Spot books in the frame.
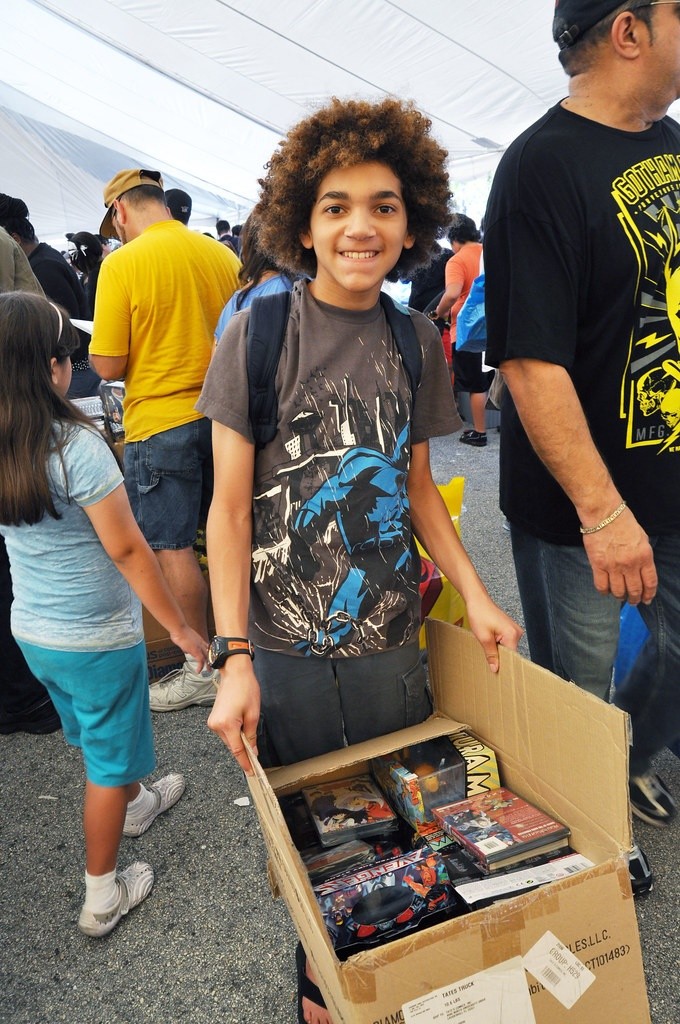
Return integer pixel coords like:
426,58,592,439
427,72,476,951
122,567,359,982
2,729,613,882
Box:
429,785,571,871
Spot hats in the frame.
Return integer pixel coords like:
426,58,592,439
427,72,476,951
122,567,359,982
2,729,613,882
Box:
216,220,229,235
68,230,102,270
553,0,628,51
98,168,164,241
165,189,192,214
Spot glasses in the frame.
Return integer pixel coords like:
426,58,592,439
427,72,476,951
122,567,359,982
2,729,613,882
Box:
105,242,112,246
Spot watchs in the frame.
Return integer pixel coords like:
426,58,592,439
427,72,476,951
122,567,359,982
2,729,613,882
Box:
206,635,255,669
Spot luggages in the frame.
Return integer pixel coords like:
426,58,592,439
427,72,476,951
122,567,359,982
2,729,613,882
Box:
457,389,501,432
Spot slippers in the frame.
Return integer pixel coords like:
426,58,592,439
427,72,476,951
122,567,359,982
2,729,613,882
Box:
295,939,328,1024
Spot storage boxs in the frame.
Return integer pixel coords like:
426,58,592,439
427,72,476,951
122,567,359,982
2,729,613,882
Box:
369,732,502,832
240,614,654,1024
143,568,216,686
315,842,458,956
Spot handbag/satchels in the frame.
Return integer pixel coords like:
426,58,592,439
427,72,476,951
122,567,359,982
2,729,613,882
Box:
455,274,488,353
417,473,474,649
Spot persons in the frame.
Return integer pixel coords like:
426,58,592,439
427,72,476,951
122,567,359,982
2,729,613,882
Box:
87,168,249,713
479,0,680,899
0,291,209,939
165,188,244,258
409,212,499,448
0,192,113,737
195,93,525,1024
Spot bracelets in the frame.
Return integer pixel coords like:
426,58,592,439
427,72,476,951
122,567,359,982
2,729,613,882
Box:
580,502,626,535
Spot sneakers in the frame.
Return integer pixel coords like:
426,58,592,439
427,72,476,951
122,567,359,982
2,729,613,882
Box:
628,766,676,898
147,662,219,712
0,694,63,736
122,773,185,838
78,861,155,938
459,431,488,447
